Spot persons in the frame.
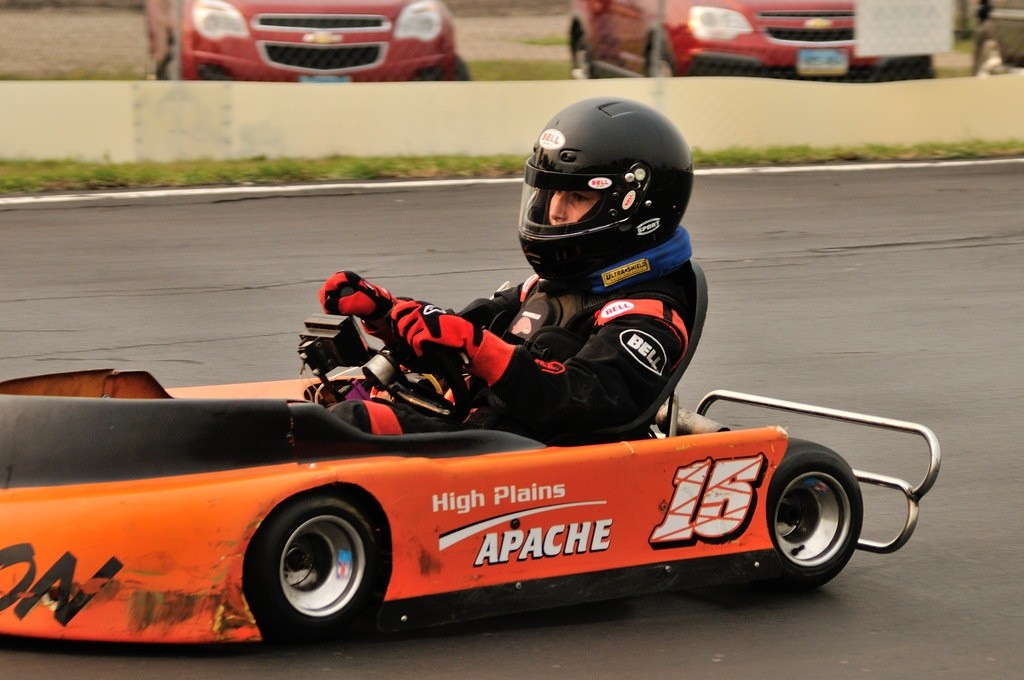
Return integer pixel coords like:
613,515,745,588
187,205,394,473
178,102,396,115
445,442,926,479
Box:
310,98,693,443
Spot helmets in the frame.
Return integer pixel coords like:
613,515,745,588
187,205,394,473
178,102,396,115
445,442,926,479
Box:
516,98,693,279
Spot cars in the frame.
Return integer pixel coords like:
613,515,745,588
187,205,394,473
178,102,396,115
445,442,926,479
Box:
142,0,474,84
972,0,1021,76
565,1,935,78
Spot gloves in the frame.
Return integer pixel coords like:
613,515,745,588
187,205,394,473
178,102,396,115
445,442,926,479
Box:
318,269,484,361
